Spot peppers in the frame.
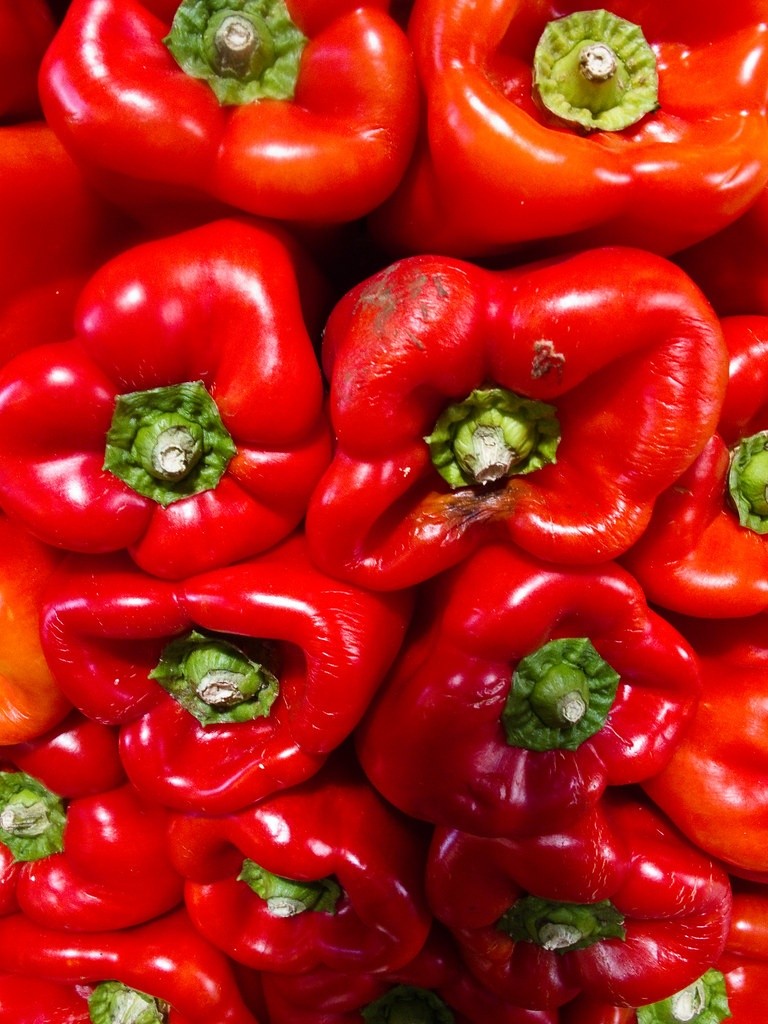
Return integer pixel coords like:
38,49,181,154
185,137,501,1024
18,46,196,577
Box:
0,0,768,1024
